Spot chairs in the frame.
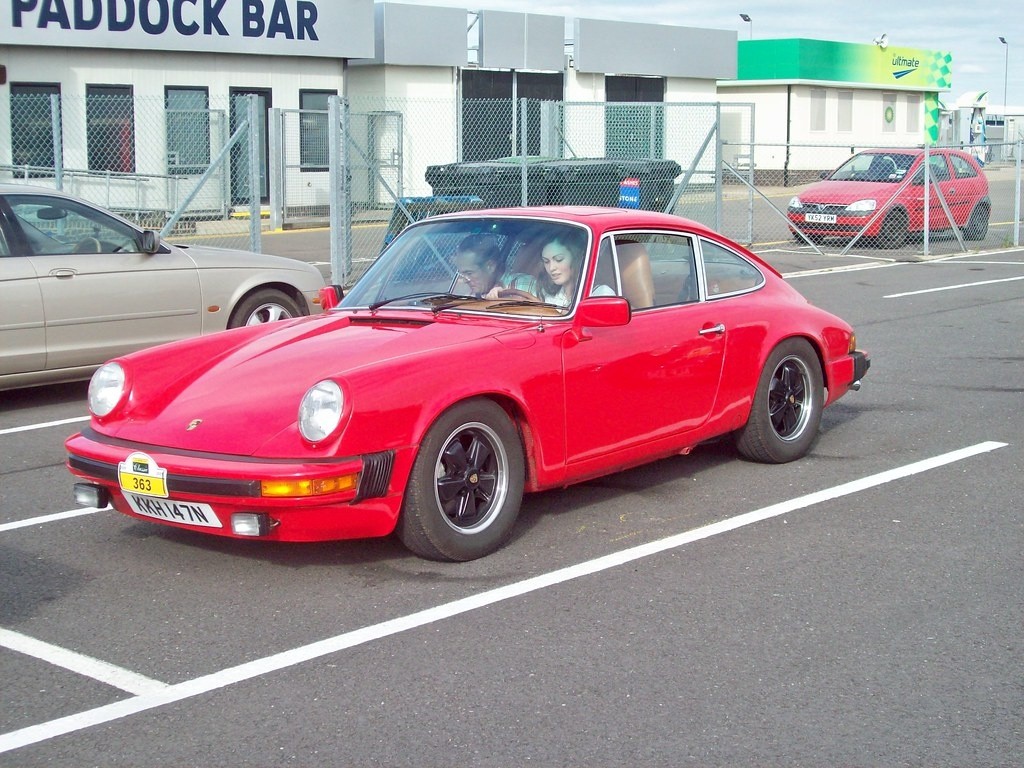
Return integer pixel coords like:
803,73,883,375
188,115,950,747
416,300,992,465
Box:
594,239,655,308
511,243,544,280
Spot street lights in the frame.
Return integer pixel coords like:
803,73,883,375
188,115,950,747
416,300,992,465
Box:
739,14,753,41
998,35,1009,107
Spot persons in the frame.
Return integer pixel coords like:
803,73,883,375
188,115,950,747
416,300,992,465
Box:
485,232,617,315
457,234,539,299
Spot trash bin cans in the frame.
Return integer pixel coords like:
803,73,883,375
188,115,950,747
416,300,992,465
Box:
426,154,683,273
383,195,488,277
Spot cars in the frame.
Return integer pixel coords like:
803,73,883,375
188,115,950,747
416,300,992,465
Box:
0,180,326,392
68,203,872,558
787,148,990,249
